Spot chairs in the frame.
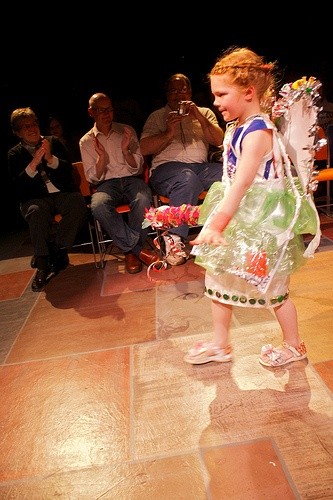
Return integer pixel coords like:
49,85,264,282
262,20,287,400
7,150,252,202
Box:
61,124,333,268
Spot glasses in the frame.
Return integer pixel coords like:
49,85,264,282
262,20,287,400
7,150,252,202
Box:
96,107,113,113
19,122,39,131
170,87,190,94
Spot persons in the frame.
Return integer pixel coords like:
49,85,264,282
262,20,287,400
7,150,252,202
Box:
141,73,225,267
145,48,323,366
47,114,79,162
7,106,83,290
79,92,166,274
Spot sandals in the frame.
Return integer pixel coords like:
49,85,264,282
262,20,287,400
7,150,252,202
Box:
183,341,235,365
257,341,308,366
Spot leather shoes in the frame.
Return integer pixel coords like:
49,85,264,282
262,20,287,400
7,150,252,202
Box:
124,251,143,274
136,237,165,270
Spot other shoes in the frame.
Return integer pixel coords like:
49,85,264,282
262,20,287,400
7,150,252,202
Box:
32,268,59,290
31,244,60,270
155,232,186,266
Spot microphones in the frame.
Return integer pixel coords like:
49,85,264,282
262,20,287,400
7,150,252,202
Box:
178,102,186,116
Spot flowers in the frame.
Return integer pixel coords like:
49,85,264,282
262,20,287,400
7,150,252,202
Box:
141,203,200,231
